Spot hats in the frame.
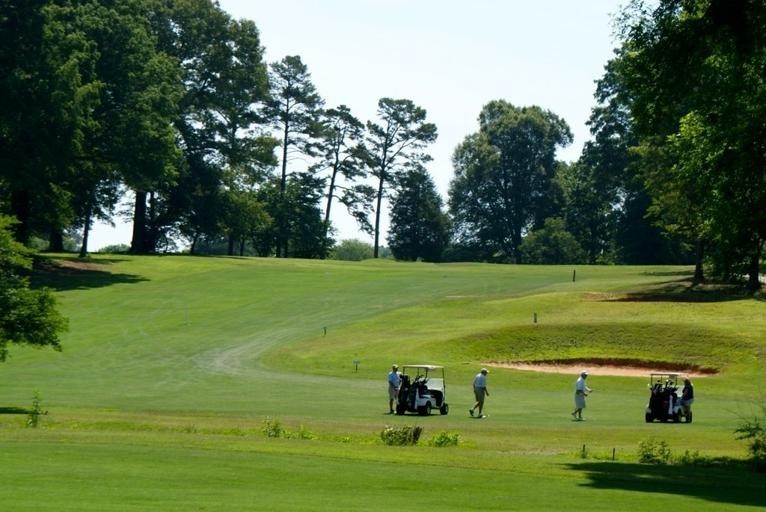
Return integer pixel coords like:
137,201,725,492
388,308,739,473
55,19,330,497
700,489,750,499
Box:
393,365,398,368
481,368,488,373
581,370,588,375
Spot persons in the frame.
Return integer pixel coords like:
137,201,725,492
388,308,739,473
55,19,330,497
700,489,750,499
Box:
468,369,490,418
570,371,594,422
387,364,403,414
680,377,693,421
